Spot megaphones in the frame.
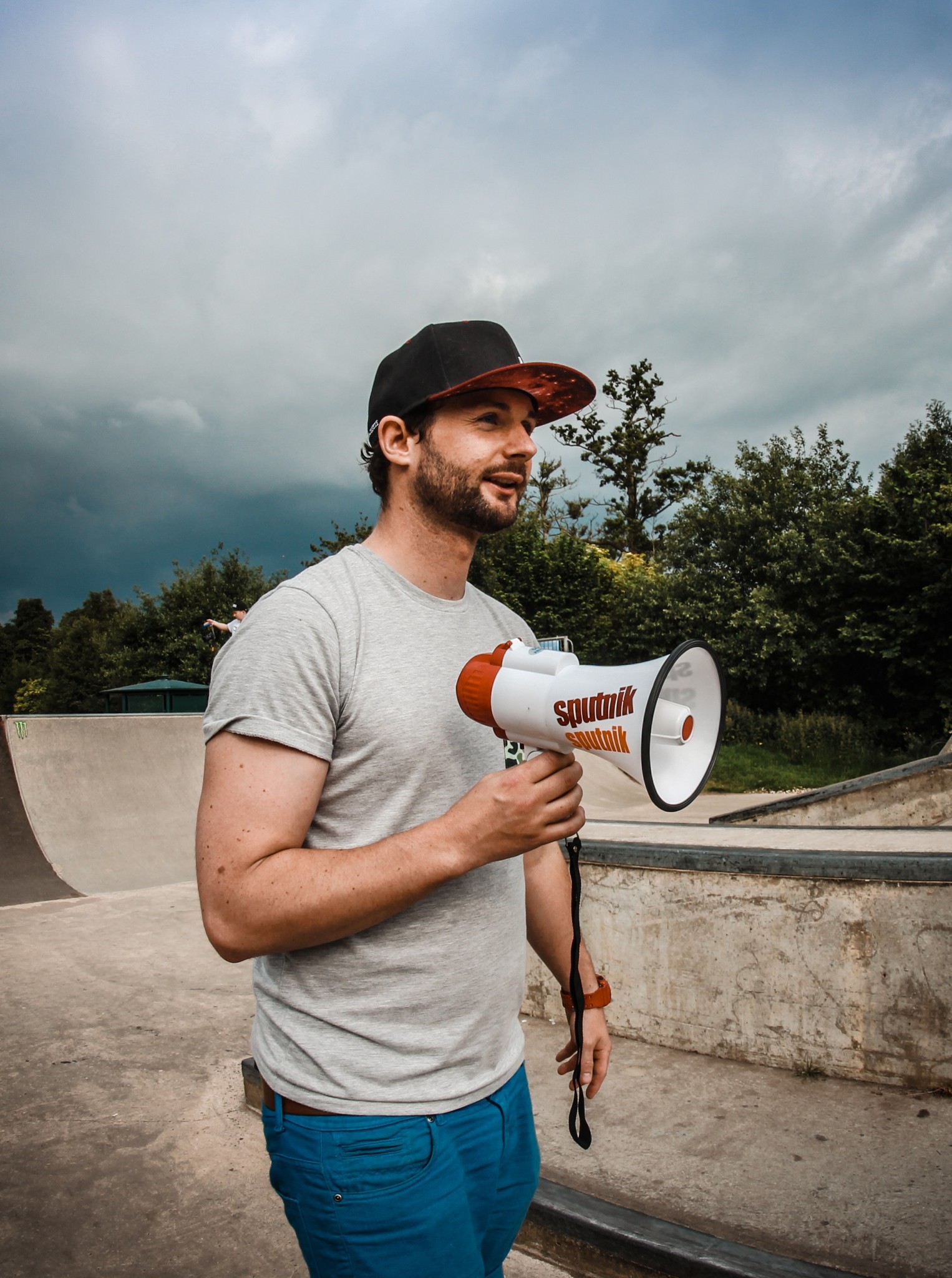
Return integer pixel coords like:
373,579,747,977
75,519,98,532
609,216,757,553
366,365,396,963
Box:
456,636,725,812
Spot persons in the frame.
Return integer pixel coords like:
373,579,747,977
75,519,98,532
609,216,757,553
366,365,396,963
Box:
206,600,249,636
195,320,613,1278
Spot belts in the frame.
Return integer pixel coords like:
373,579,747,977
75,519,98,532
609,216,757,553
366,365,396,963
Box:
262,1077,340,1116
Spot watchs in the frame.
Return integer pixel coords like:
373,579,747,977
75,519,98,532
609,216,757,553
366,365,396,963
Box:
560,973,612,1010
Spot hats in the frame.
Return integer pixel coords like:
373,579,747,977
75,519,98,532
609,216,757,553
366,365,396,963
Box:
367,321,596,455
